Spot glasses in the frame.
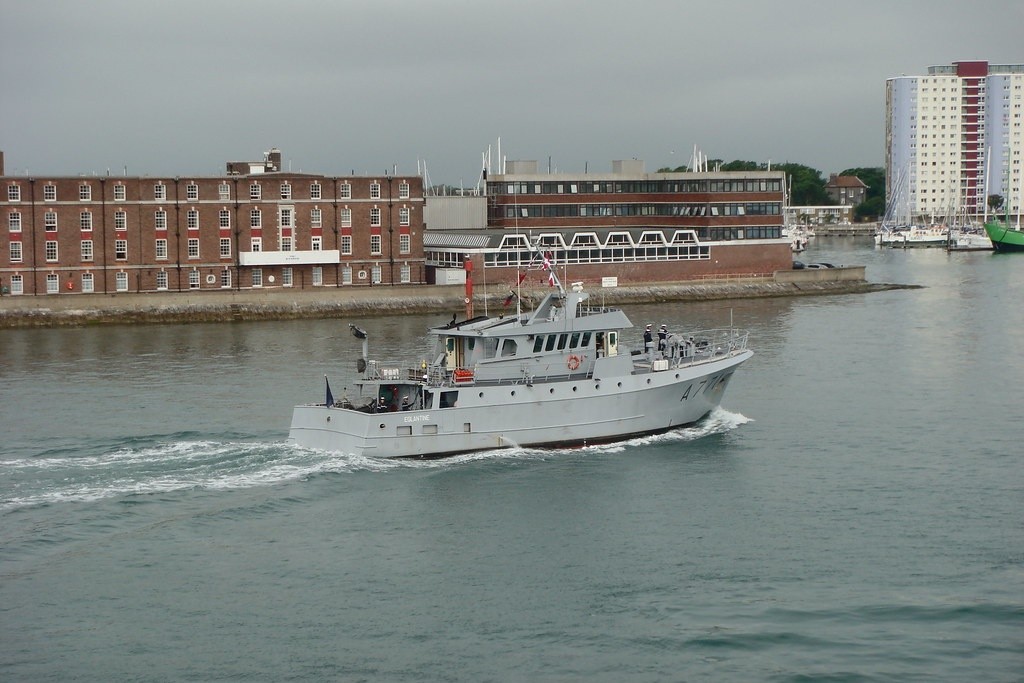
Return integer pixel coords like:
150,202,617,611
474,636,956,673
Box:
406,399,408,400
380,399,385,400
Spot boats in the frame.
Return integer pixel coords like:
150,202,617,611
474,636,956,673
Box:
982,148,1024,252
781,216,815,252
872,146,993,251
288,184,755,459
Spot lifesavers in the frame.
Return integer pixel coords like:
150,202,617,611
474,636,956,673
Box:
567,356,580,370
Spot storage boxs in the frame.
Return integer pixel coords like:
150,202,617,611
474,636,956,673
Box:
381,369,399,380
653,360,668,371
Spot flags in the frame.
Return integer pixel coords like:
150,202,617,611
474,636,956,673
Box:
516,274,525,286
549,271,554,287
503,296,512,306
325,378,333,408
543,249,550,271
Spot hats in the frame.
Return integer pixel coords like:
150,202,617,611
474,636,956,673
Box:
661,325,666,326
646,324,653,327
403,396,408,399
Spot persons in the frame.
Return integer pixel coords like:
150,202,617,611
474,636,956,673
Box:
658,324,668,351
643,324,652,353
